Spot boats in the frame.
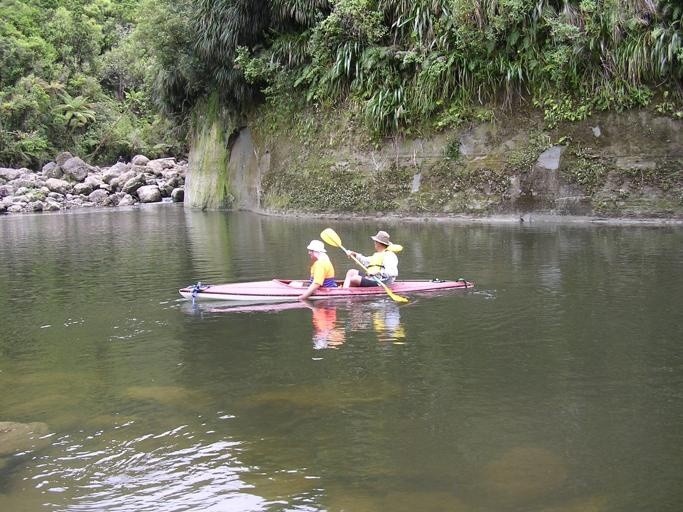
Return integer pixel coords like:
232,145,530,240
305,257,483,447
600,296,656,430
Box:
178,278,476,304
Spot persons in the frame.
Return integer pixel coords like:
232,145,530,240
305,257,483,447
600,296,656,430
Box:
341,230,404,289
295,239,336,302
349,298,405,345
301,299,337,351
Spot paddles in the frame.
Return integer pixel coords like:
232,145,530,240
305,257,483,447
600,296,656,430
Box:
320,227,408,302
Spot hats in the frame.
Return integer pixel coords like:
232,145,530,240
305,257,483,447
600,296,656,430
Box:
307,240,327,253
370,231,390,245
